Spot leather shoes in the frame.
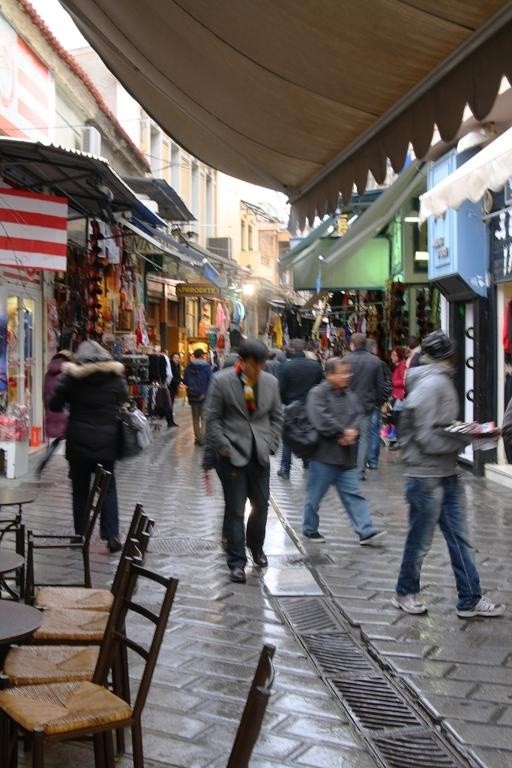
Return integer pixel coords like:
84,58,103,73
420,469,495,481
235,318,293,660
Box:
71,536,88,547
247,542,267,565
230,567,245,582
277,468,289,479
109,535,121,551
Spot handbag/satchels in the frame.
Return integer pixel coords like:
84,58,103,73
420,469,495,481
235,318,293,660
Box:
278,386,328,459
115,400,150,454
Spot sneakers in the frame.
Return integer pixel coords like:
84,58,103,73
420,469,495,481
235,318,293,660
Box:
359,530,386,545
391,593,428,614
303,531,324,543
455,597,507,617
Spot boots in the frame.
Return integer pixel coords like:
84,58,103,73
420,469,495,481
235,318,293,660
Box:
33,446,52,482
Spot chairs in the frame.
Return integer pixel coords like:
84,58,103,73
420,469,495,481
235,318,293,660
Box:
32,518,154,708
28,464,112,588
3,538,142,754
1,558,179,768
225,643,277,767
32,501,147,609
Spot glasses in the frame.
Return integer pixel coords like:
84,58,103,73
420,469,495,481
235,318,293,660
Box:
328,370,353,377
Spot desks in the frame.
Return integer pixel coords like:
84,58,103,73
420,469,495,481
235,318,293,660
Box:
1,546,25,600
0,599,44,643
1,488,34,526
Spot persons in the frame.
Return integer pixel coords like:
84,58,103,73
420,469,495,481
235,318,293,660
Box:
193,327,346,360
279,338,323,480
302,358,387,542
186,351,214,449
200,339,284,582
394,332,508,618
340,335,384,479
48,342,134,553
166,354,179,405
367,340,391,470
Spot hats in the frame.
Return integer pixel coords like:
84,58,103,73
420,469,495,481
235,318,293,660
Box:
420,330,459,360
73,340,112,362
193,349,206,355
